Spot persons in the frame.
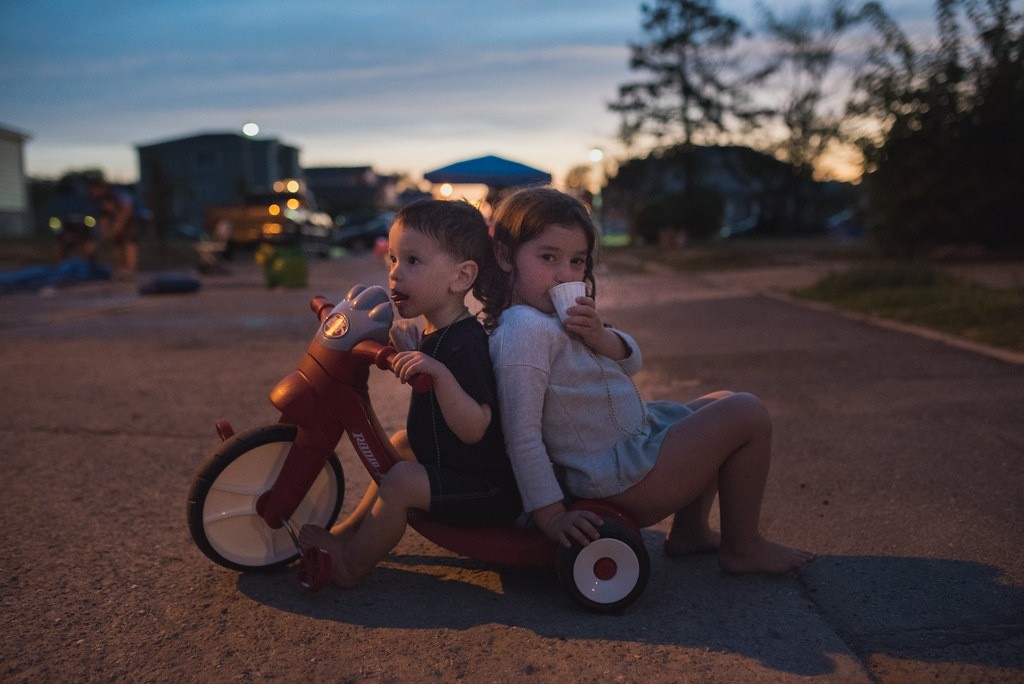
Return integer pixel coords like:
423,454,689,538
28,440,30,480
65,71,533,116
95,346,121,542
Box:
474,187,817,573
298,199,523,589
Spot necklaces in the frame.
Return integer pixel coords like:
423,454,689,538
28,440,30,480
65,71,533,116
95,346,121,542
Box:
430,306,468,469
551,312,647,434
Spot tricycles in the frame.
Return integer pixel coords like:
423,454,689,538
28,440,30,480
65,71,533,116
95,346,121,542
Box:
181,284,651,616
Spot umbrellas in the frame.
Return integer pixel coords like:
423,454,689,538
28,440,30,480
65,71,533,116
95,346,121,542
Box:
424,156,552,222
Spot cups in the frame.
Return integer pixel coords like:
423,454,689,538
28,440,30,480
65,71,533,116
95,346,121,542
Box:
548,281,587,324
